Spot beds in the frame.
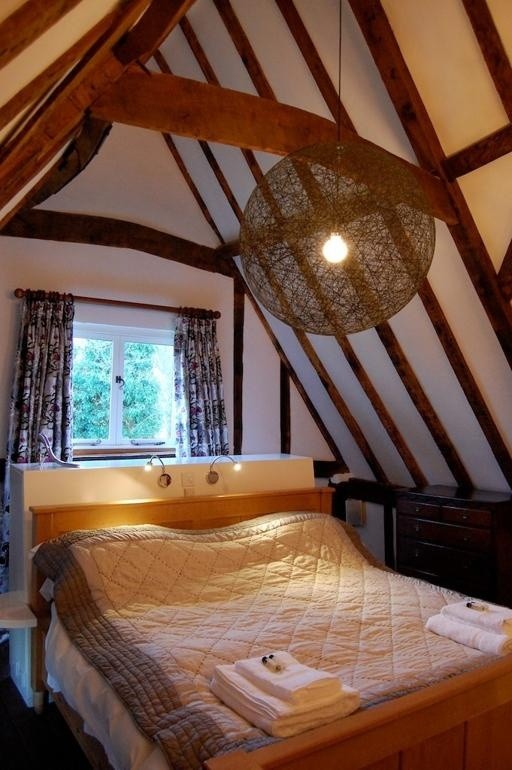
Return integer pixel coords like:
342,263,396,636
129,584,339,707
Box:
30,487,512,769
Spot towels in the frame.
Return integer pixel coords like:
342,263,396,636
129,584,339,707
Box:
441,600,512,633
209,653,360,738
425,600,512,657
234,650,341,704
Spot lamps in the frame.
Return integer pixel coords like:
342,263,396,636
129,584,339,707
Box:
144,455,172,488
238,0,436,336
206,455,242,484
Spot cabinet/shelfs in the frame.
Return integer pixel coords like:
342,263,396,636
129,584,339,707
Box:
394,485,512,605
